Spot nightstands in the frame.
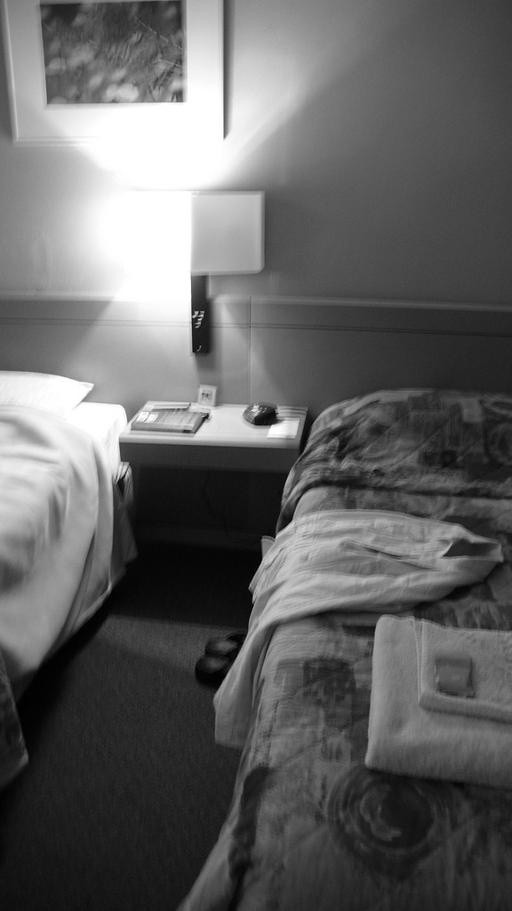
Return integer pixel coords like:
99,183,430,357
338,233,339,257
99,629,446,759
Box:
119,401,309,560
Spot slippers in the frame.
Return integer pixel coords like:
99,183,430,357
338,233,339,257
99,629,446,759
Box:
196,632,248,688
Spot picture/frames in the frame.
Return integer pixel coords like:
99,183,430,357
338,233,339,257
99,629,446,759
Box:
1,0,225,145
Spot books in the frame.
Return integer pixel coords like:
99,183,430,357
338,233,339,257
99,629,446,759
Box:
129,409,210,435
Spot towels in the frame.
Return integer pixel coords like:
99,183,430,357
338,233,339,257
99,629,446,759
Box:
363,612,511,790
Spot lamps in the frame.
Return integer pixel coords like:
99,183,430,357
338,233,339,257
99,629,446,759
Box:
190,192,266,356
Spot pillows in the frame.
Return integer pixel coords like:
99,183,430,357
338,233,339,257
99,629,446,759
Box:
1,369,95,422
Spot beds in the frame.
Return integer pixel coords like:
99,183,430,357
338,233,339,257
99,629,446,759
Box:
234,387,512,910
0,367,127,779
